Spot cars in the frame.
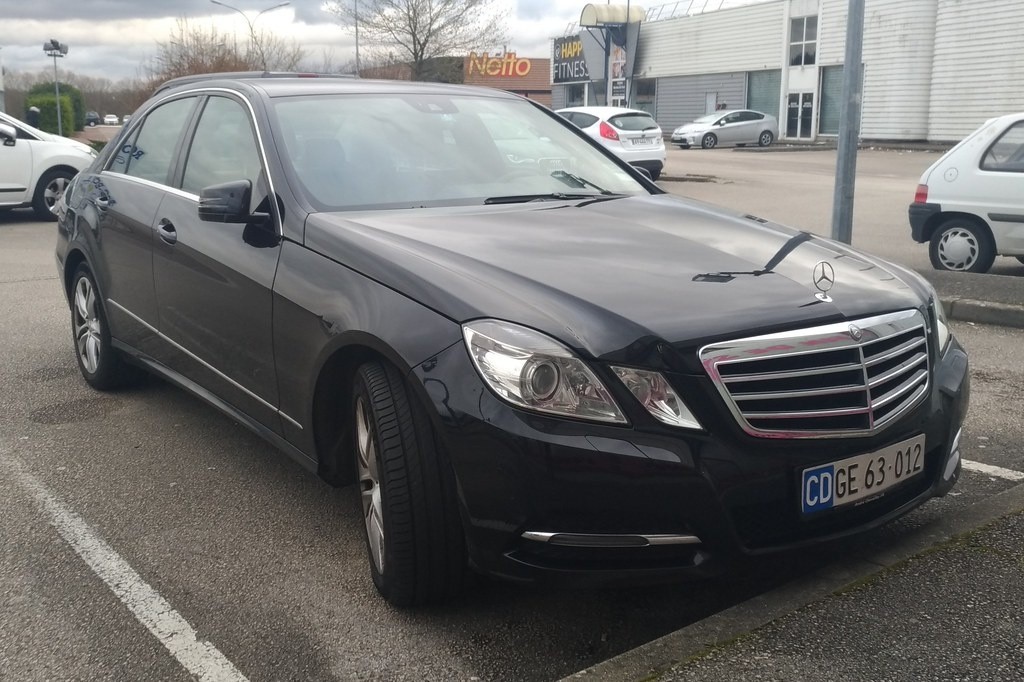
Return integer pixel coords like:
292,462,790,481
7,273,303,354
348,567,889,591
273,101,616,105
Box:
57,70,970,611
84,110,100,126
908,111,1024,276
103,114,119,125
0,109,101,222
669,109,780,150
554,106,666,182
123,114,130,123
